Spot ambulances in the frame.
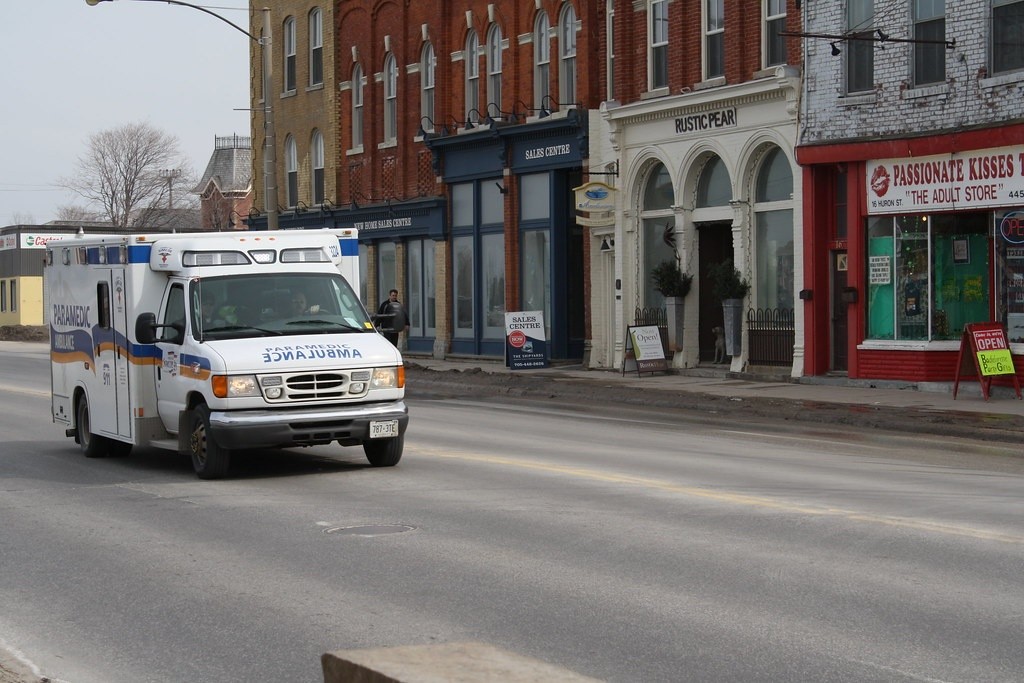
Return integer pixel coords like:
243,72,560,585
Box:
41,225,409,482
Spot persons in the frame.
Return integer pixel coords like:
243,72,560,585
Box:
374,289,410,348
176,293,232,333
287,291,322,318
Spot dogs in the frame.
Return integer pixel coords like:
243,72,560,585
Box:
712,327,731,364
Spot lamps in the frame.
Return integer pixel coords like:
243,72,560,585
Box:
465,109,508,130
830,29,890,56
670,205,692,214
246,207,262,226
293,201,309,220
729,199,751,210
485,103,526,125
539,95,582,118
417,116,456,136
601,234,614,250
441,114,479,137
350,192,389,211
389,191,426,217
511,101,552,125
78,228,85,238
319,198,352,216
229,211,250,228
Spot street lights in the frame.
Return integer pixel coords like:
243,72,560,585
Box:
83,0,280,231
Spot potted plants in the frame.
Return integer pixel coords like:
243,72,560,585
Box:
708,257,752,356
651,259,695,353
